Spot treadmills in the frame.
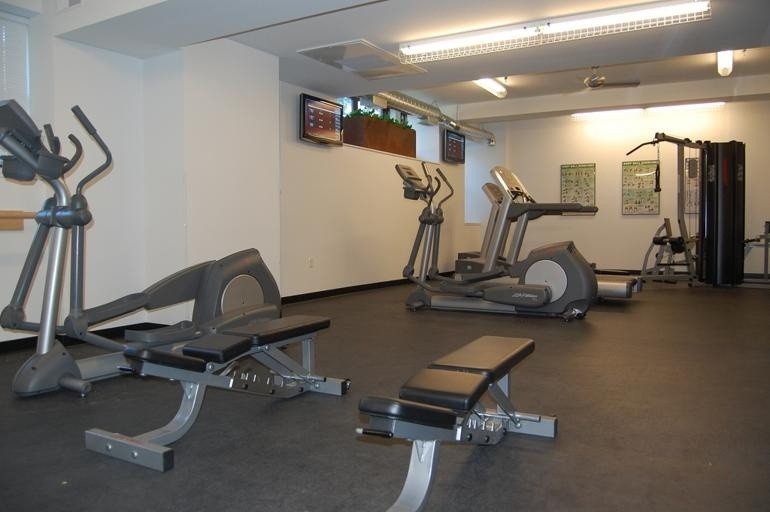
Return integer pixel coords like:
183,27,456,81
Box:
454,178,643,303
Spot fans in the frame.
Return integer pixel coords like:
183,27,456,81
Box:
564,74,641,96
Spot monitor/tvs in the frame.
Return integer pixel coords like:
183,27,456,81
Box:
444,128,465,163
300,93,343,146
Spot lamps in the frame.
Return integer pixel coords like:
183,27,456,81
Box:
400,0,710,55
473,78,507,99
716,50,732,76
